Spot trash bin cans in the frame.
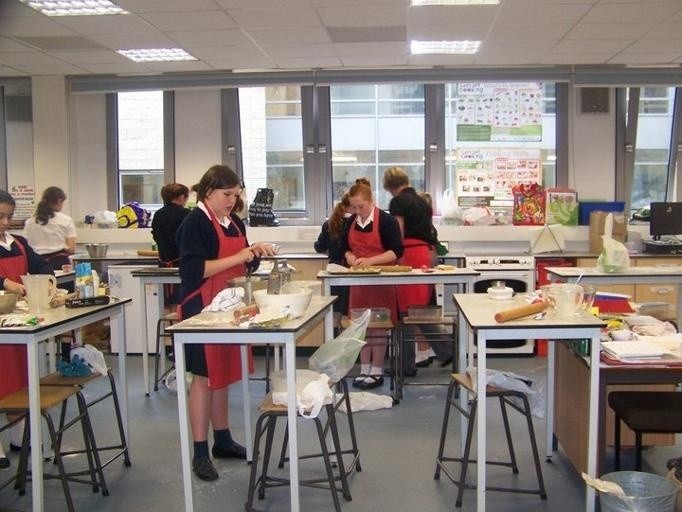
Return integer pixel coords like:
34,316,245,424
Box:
579,469,681,511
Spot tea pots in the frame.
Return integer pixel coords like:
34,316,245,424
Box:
19,275,57,312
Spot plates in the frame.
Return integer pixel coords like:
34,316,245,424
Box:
327,268,382,275
129,267,181,276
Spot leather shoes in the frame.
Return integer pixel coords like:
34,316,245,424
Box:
212,444,247,462
416,360,430,367
0,457,11,471
9,441,45,455
385,367,418,378
191,459,220,484
352,372,366,387
359,376,385,390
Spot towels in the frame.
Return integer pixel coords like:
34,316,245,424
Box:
199,286,245,313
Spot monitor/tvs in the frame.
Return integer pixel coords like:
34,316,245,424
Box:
649,201,682,241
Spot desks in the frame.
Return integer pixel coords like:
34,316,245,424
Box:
534,251,682,320
48,270,101,375
452,293,608,512
318,269,481,409
438,250,467,317
163,296,339,511
0,291,132,512
72,254,159,270
545,266,682,333
555,340,682,511
130,267,293,397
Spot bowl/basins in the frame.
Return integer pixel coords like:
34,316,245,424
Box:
227,276,262,300
0,290,21,314
85,243,109,258
539,284,597,317
253,289,313,318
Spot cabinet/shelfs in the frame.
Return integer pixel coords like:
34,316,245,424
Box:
259,260,329,347
108,265,167,354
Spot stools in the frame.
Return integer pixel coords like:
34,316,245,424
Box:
608,391,682,472
0,387,100,512
39,368,132,494
245,378,361,511
154,313,181,391
398,316,458,400
340,315,400,403
435,374,547,508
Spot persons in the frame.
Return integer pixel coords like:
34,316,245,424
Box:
315,190,352,338
382,167,454,377
176,165,275,480
336,178,404,390
151,183,192,361
415,194,437,367
24,187,77,364
0,191,57,471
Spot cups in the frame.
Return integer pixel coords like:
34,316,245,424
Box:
491,280,507,288
